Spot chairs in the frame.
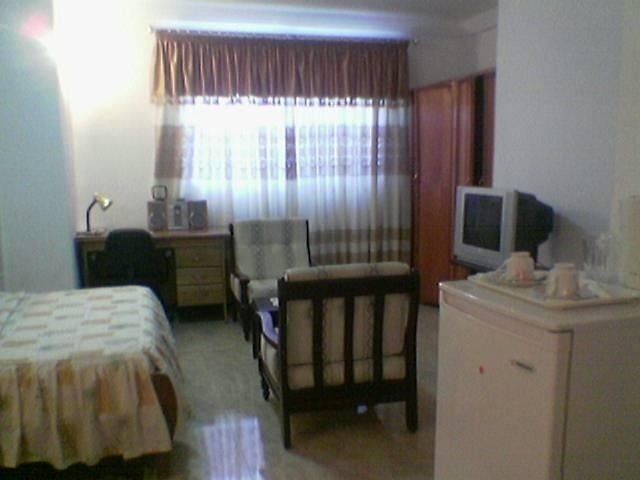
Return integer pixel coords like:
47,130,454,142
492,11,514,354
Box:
86,228,168,301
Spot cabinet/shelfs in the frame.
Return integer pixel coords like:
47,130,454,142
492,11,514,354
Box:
73,224,229,321
228,217,312,358
410,68,496,306
246,261,421,450
0,285,183,471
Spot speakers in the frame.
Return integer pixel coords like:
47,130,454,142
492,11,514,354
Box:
146,200,168,230
187,200,209,231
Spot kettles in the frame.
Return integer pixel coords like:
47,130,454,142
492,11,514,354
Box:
609,194,640,291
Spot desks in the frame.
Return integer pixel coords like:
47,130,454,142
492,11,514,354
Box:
251,298,281,358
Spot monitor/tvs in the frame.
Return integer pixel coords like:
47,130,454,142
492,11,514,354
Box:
450,185,553,274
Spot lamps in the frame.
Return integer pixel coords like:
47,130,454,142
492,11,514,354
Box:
80,193,113,235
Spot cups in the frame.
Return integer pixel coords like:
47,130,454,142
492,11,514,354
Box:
497,251,536,284
545,262,579,300
579,231,616,282
